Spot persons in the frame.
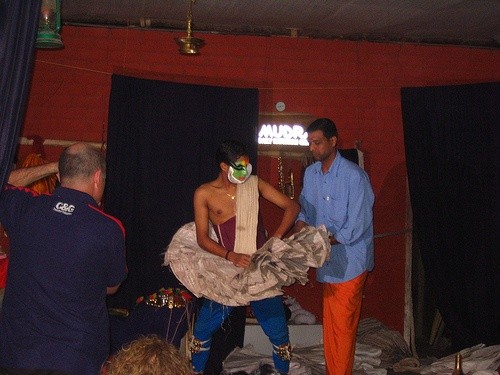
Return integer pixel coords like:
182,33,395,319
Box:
101,336,193,375
293,117,377,375
0,142,128,375
165,138,331,375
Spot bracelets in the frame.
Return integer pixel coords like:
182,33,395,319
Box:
225,250,232,260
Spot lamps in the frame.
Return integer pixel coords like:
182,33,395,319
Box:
36,0,64,50
